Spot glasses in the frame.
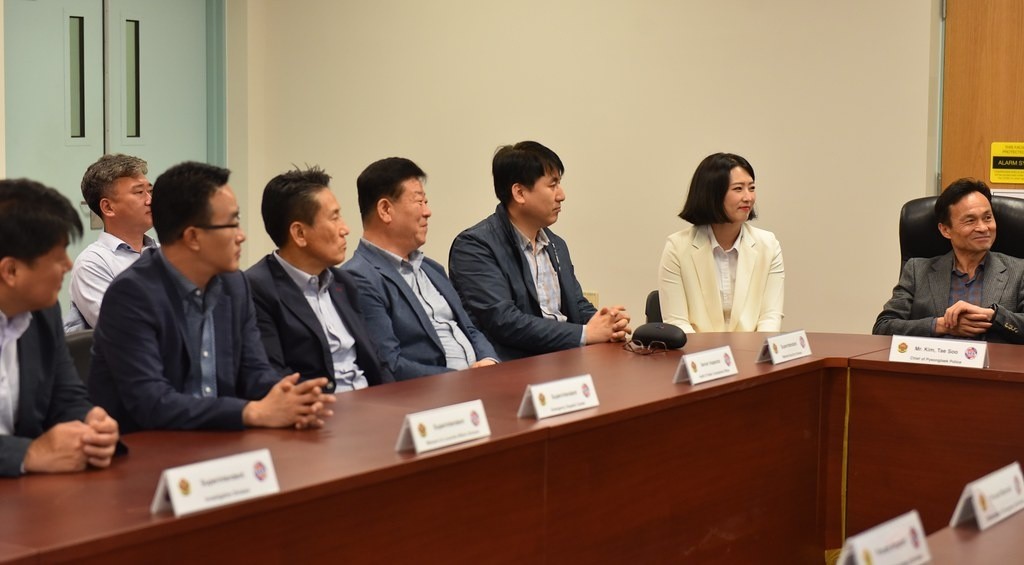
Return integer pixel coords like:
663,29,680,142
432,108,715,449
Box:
176,217,242,238
622,340,669,355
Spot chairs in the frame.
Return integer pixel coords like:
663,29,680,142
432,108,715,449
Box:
898,195,1024,278
65,330,92,387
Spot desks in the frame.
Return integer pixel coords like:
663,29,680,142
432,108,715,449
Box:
1,331,1024,564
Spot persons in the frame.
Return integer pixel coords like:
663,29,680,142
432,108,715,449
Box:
449,140,631,362
872,178,1024,345
64,153,161,334
659,153,784,333
0,177,128,478
340,156,503,381
85,160,337,435
244,162,394,396
